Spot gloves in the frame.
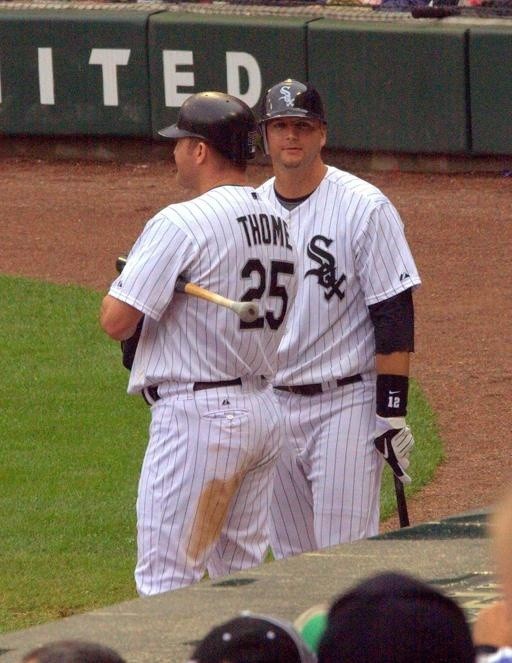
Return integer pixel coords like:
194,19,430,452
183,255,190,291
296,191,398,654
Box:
371,411,417,490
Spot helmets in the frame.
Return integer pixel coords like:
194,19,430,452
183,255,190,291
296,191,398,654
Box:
253,76,330,128
156,89,258,161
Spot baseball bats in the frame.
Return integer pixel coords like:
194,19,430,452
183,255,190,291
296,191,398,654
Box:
113,249,258,322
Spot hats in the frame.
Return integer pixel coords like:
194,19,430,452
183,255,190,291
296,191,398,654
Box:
291,601,332,656
317,569,476,663
188,609,301,662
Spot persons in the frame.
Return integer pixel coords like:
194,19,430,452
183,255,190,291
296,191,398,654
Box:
98,90,297,599
253,79,421,555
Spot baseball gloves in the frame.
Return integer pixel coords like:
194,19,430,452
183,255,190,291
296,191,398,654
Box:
121,316,145,370
